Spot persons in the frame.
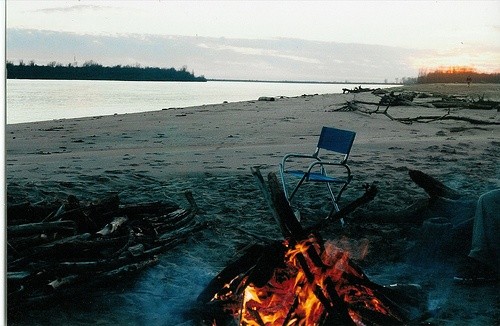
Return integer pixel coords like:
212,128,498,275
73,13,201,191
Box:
463,188,500,282
54,195,97,235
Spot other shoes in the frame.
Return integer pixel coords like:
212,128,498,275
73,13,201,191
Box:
454,261,497,282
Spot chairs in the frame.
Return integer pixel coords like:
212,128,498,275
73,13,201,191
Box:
278,126,357,230
